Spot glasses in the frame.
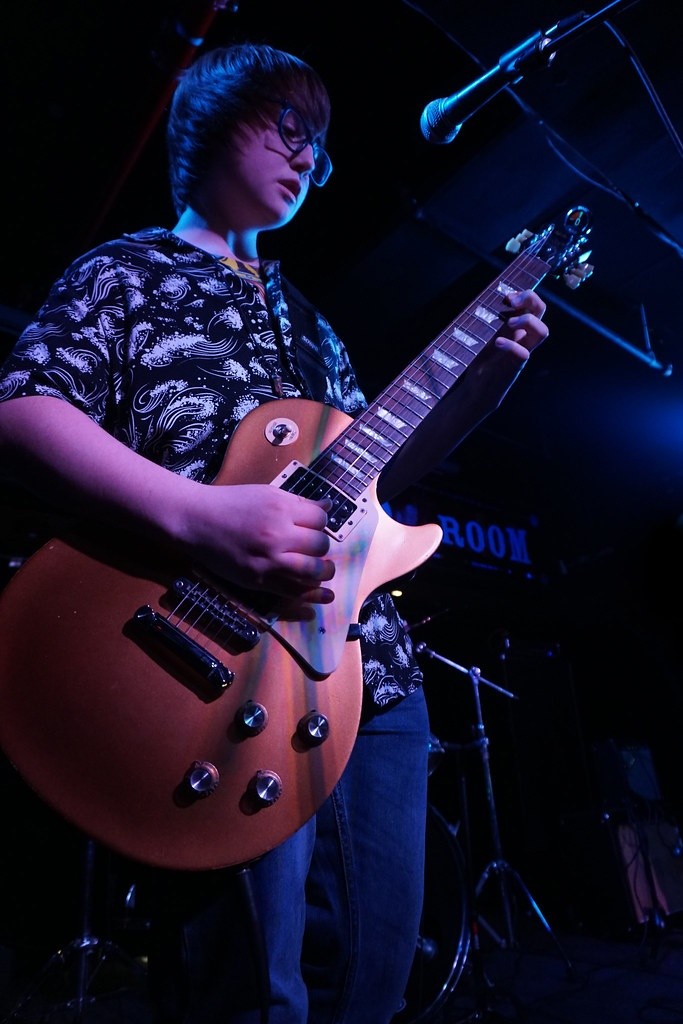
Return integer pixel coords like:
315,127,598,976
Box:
272,108,334,186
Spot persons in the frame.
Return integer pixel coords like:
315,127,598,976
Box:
0,43,548,1024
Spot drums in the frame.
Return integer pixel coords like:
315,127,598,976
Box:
147,801,473,1024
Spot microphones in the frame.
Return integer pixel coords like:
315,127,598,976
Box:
420,9,590,144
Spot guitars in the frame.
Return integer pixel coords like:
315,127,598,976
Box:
0,194,601,902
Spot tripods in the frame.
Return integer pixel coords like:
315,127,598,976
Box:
0,835,154,1024
413,641,577,975
